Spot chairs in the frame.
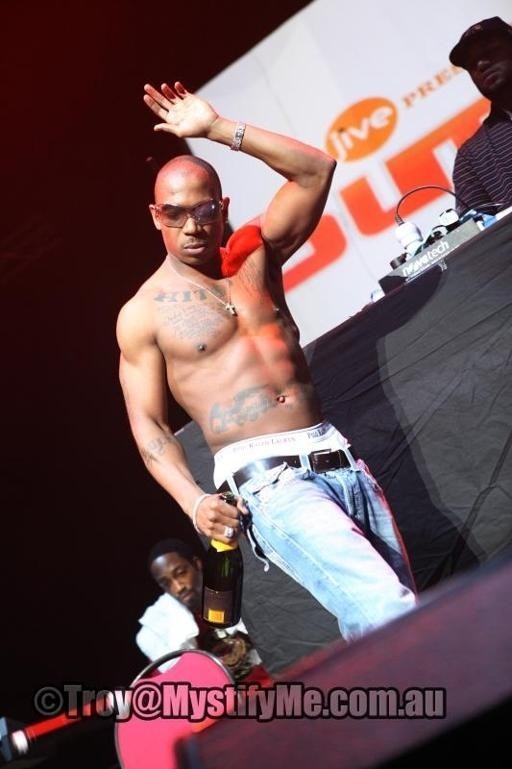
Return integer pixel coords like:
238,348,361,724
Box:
114,649,243,769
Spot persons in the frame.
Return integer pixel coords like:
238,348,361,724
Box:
133,535,274,710
448,13,512,220
114,76,425,642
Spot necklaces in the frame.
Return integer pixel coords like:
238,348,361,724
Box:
164,250,238,317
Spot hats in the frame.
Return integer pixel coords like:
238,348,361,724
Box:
449,16,512,66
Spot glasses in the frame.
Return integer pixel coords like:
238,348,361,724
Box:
156,199,223,228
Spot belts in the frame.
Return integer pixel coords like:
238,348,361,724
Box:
216,445,360,500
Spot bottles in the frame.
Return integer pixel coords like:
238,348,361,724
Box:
200,491,244,629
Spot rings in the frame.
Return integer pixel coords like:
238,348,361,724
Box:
223,526,235,539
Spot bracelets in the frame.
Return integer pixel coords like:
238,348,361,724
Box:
228,118,246,153
191,493,211,536
